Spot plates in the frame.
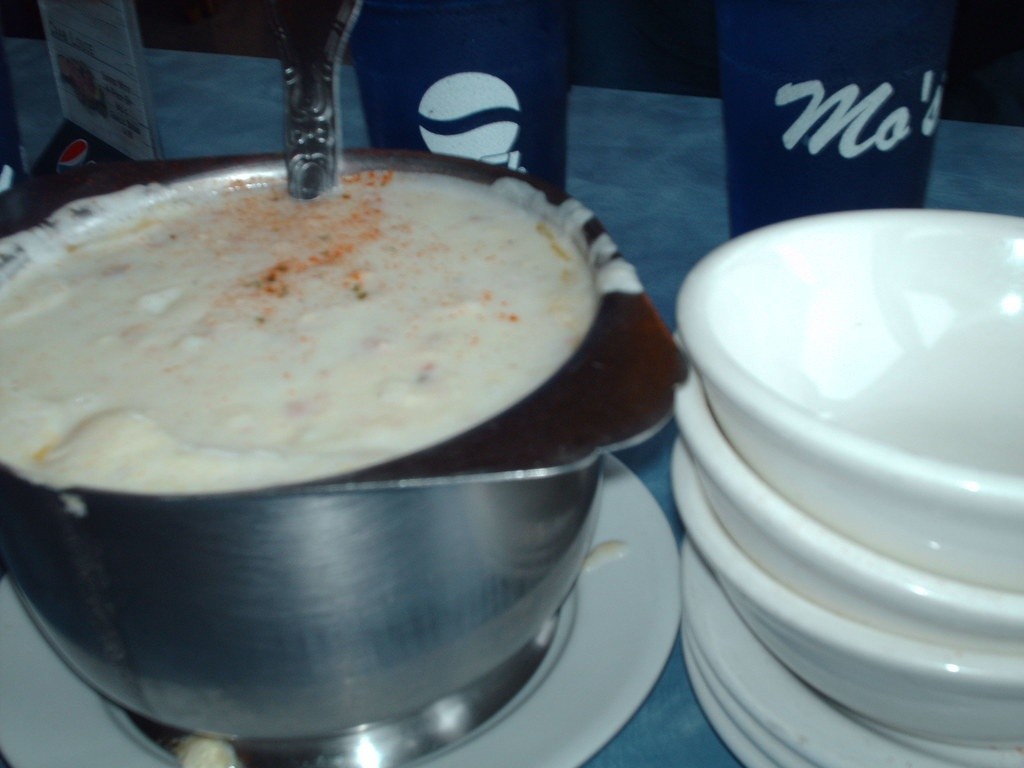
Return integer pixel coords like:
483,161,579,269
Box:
0,450,680,768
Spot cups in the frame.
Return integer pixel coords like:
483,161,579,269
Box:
0,155,683,751
714,0,953,236
349,0,569,195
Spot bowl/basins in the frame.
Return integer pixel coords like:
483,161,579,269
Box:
678,204,1024,768
